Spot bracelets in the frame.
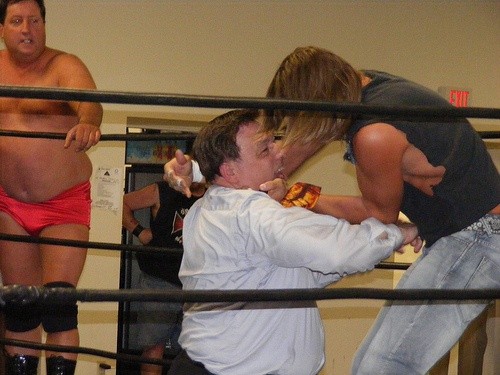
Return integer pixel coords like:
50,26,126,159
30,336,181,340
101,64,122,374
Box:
132,224,144,237
191,159,203,183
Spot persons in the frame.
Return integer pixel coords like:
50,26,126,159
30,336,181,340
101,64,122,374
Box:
168,106,424,375
122,151,208,375
0,0,103,375
162,46,499,374
